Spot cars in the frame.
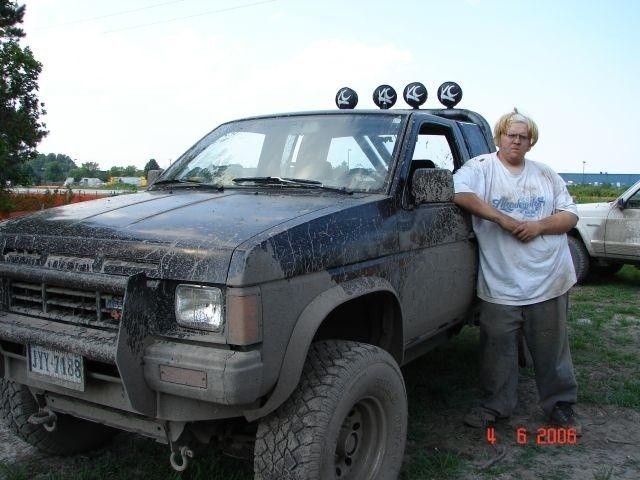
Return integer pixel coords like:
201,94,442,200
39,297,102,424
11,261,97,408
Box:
566,179,639,282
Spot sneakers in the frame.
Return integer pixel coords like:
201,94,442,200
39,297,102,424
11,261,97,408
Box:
465,406,501,427
553,400,584,437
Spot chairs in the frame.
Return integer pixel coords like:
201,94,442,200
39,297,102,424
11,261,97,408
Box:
408,157,435,193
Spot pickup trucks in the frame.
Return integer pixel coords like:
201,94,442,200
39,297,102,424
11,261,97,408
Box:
0,80,497,480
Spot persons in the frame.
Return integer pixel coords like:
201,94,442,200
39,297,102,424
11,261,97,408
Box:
449,111,581,437
